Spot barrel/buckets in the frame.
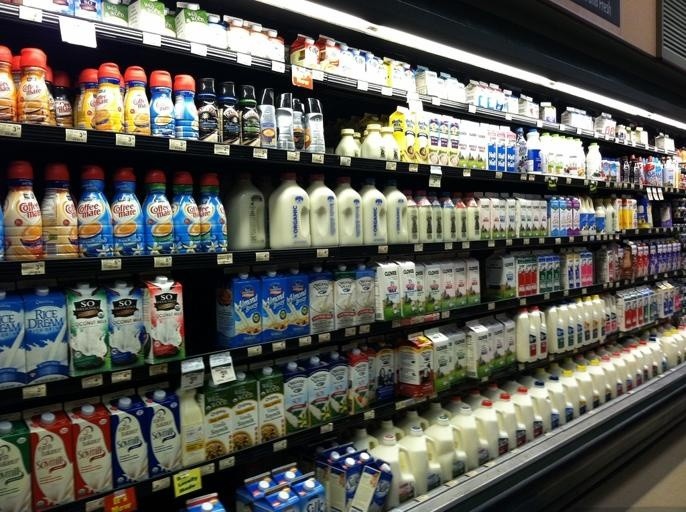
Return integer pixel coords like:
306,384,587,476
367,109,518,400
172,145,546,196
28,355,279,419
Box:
600,294,612,335
637,341,653,382
584,295,600,343
446,396,471,412
572,364,594,412
398,425,441,495
492,393,526,450
423,414,468,481
660,330,678,370
627,343,644,387
304,172,338,248
520,374,539,388
620,348,637,389
670,328,685,364
575,296,592,346
529,379,560,431
503,379,523,393
558,299,576,351
399,410,430,433
534,368,552,381
424,403,451,422
226,173,265,249
530,304,547,359
608,350,627,395
562,357,578,370
606,292,617,332
451,405,489,469
545,294,567,353
370,434,416,510
559,368,580,423
485,383,508,401
592,293,606,341
350,427,379,451
376,419,405,444
473,400,509,458
566,298,584,348
547,362,564,377
513,307,538,363
359,178,387,245
512,385,544,442
599,354,617,399
586,358,606,405
333,176,363,246
381,179,408,244
646,337,663,376
574,354,589,366
269,172,311,250
468,389,487,409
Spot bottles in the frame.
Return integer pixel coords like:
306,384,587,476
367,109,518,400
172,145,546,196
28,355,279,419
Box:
173,74,198,140
97,63,125,133
0,198,5,261
354,133,362,149
239,86,259,147
199,78,217,143
1,45,23,122
259,88,279,151
123,66,147,136
428,191,443,243
171,170,202,254
304,99,326,153
143,169,171,254
200,172,229,252
278,94,295,152
77,165,111,257
464,192,480,241
452,192,468,242
362,123,380,160
112,169,145,256
402,189,419,243
40,164,77,257
4,161,42,261
18,49,48,125
334,128,359,156
605,198,617,234
74,70,96,130
51,73,74,128
525,128,542,172
414,189,434,243
587,143,602,179
540,133,586,176
219,84,241,145
294,99,309,154
516,127,528,172
149,69,173,136
382,127,400,161
440,192,457,243
594,198,606,233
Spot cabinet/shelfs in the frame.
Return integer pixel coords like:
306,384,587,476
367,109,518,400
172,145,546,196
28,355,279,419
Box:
0,0,686,512
